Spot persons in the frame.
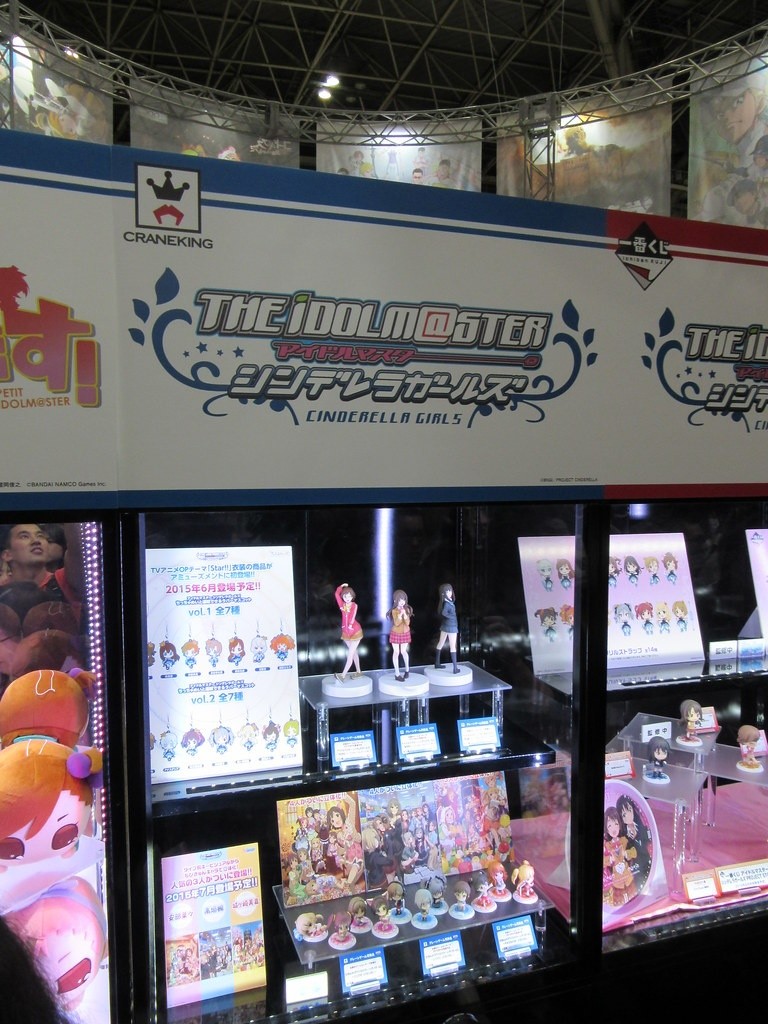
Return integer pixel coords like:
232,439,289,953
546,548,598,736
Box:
647,736,673,779
415,888,432,921
348,897,368,927
333,583,363,684
326,912,353,943
0,523,86,622
487,861,507,894
294,912,327,936
421,875,447,908
467,869,493,906
366,891,391,932
454,881,469,911
511,860,534,897
678,699,703,741
736,725,761,766
384,880,406,917
384,590,414,682
435,583,460,674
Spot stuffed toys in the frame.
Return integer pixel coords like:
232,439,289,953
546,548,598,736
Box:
0,631,110,1024
